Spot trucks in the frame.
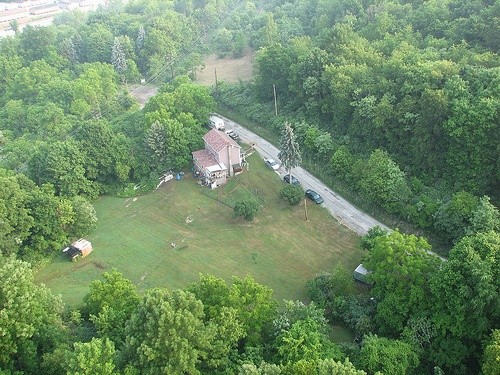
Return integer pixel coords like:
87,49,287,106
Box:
354,264,377,288
209,117,225,131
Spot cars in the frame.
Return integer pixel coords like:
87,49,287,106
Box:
226,129,238,140
264,158,279,171
306,189,323,204
284,175,301,188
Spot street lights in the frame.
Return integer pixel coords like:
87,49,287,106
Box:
272,84,277,116
